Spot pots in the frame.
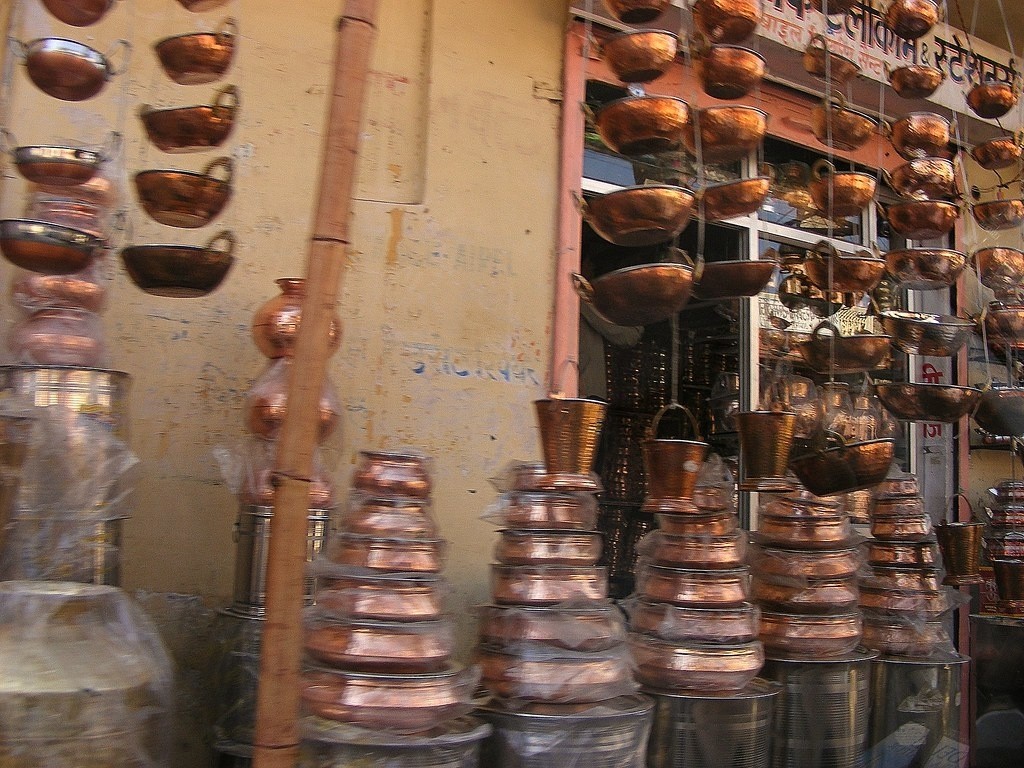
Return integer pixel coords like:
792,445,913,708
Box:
690,31,767,99
122,232,235,298
804,0,1024,240
136,158,233,227
572,256,704,327
42,0,112,26
156,19,236,84
571,183,704,247
0,213,124,274
602,0,671,22
673,249,776,299
0,127,122,186
684,0,764,43
582,94,696,155
141,85,237,154
6,36,131,101
788,240,1024,496
682,104,768,164
694,165,775,222
588,29,686,83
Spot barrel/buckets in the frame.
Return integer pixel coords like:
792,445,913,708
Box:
991,532,1024,600
870,652,971,768
296,714,493,767
732,412,796,479
0,622,168,767
762,647,880,768
0,365,127,596
232,506,332,617
643,404,707,498
534,359,607,473
214,607,265,745
935,494,984,576
642,674,784,768
474,690,656,767
